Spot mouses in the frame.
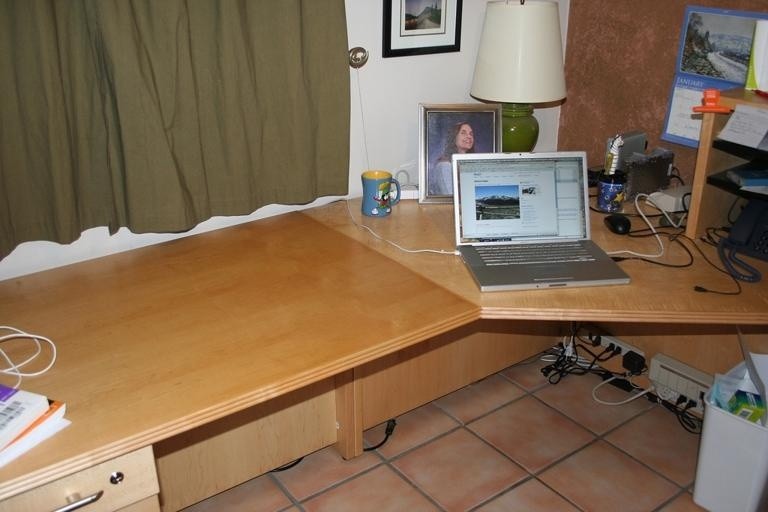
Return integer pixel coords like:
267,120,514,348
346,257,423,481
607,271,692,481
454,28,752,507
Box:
604,215,630,234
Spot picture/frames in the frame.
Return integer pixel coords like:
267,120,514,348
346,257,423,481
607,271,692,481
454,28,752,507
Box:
413,102,500,205
380,0,463,59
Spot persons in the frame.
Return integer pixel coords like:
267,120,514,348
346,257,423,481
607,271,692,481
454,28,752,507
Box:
430,121,475,195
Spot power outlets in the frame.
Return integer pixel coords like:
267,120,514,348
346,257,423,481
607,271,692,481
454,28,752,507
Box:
646,353,714,416
576,324,644,361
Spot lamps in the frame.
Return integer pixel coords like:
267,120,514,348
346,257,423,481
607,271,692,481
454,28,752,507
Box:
466,0,569,152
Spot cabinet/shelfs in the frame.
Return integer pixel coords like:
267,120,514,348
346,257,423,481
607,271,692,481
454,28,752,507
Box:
682,82,767,243
0,443,162,510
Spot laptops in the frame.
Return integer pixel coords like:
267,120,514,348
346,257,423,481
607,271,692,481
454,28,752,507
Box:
451,151,631,292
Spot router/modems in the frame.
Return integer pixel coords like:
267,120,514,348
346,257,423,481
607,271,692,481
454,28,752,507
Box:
645,185,692,212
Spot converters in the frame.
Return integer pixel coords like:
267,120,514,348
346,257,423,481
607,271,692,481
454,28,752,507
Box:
623,351,646,373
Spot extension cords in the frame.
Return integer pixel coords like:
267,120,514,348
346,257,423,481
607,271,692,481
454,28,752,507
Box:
648,352,714,406
581,325,646,366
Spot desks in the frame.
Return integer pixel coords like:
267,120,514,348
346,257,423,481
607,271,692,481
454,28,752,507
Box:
1,192,768,510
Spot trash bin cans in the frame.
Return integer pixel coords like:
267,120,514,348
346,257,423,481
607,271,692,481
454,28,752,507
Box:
693,353,767,511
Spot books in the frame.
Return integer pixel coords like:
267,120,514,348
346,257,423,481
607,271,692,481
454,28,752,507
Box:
0,383,72,470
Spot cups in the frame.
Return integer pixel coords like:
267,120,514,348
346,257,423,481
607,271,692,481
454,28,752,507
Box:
600,182,625,214
360,172,402,218
